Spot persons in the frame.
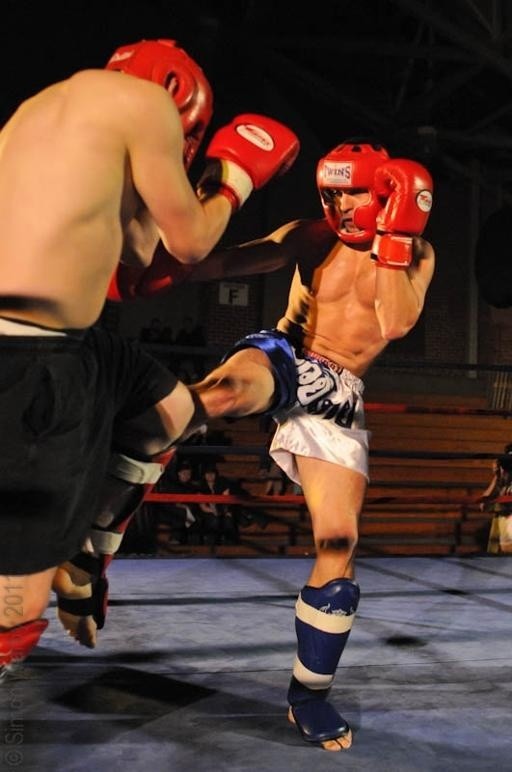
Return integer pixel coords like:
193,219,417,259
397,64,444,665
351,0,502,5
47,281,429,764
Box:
141,317,306,555
183,137,436,751
0,37,302,688
479,444,512,554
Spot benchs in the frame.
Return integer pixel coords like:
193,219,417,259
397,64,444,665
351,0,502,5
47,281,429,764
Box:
354,408,510,554
156,410,315,553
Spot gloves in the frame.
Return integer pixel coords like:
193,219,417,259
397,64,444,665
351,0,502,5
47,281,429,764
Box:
371,159,437,268
205,109,301,200
